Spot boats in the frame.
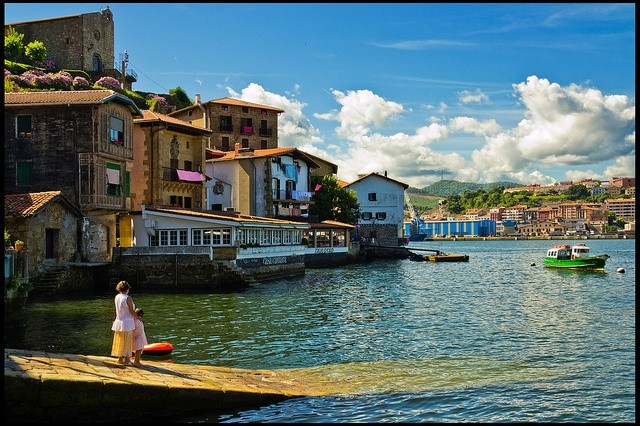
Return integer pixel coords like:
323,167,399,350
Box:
543,243,610,271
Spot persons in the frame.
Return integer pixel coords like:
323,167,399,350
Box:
110,281,143,368
134,307,148,368
